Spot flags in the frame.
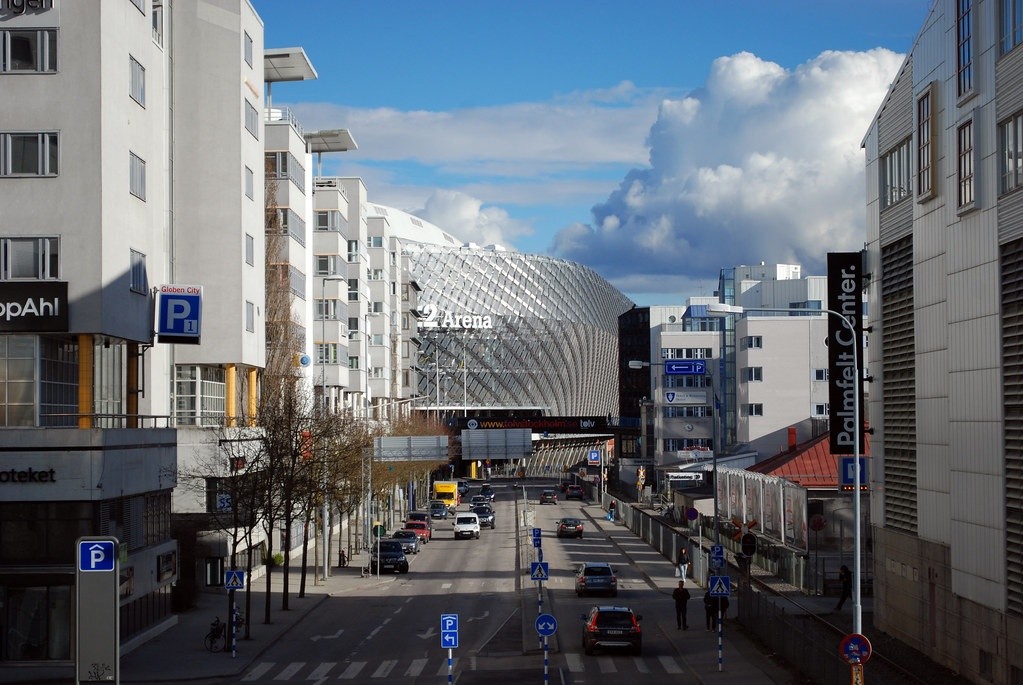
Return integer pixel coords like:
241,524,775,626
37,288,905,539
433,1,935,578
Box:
418,349,436,365
455,349,464,368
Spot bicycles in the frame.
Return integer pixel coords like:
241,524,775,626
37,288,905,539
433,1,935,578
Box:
204,606,248,653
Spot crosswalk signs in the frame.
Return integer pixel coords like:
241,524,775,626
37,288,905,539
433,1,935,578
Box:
531,562,548,580
226,572,244,589
710,576,731,596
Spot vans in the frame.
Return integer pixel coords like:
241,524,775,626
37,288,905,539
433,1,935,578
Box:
452,512,480,540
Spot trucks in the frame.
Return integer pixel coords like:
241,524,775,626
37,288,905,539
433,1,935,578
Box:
432,481,457,514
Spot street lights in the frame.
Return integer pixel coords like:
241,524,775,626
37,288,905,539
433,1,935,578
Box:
413,350,424,512
423,334,467,426
364,312,379,578
322,274,345,580
705,303,861,684
628,360,720,546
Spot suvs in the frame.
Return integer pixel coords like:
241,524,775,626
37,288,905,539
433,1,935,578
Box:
572,562,618,597
579,605,642,656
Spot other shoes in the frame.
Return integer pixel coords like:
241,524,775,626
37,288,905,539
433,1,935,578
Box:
712,630,715,632
706,629,710,632
682,625,689,630
678,627,681,630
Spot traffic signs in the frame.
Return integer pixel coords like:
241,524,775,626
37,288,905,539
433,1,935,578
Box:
666,361,704,375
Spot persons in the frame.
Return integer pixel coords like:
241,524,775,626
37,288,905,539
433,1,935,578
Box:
209,616,224,639
341,550,346,566
609,500,616,521
672,580,690,631
607,413,613,426
835,565,853,609
678,548,691,582
704,589,729,632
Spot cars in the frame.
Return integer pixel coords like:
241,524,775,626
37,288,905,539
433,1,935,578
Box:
540,490,558,505
555,518,584,539
457,479,496,530
368,500,449,574
560,481,583,501
